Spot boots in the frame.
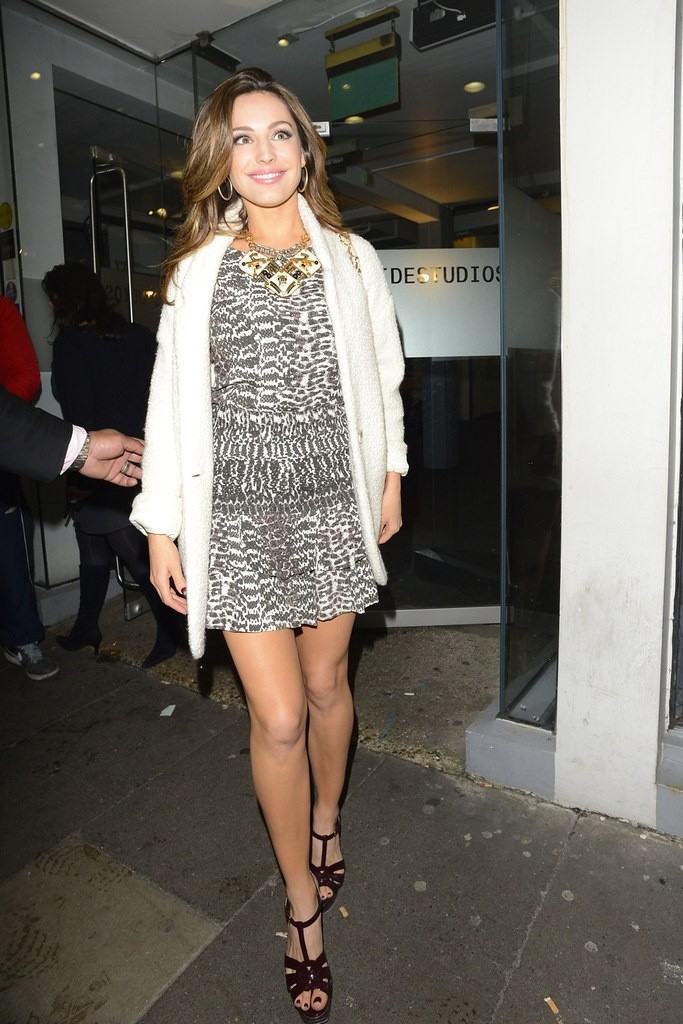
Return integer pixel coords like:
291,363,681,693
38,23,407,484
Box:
138,582,184,668
57,565,110,659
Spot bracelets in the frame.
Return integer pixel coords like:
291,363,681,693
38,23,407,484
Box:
67,430,91,473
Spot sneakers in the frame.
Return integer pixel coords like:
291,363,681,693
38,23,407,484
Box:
0,642,59,681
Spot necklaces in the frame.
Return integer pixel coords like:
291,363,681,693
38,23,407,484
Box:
242,229,311,257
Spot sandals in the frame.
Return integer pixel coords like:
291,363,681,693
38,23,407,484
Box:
284,870,333,1024
309,814,345,912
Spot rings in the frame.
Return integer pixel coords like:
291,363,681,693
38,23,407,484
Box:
121,462,130,473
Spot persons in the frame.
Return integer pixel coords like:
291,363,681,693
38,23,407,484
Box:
129,66,411,1024
41,261,186,672
0,295,60,680
0,382,146,488
